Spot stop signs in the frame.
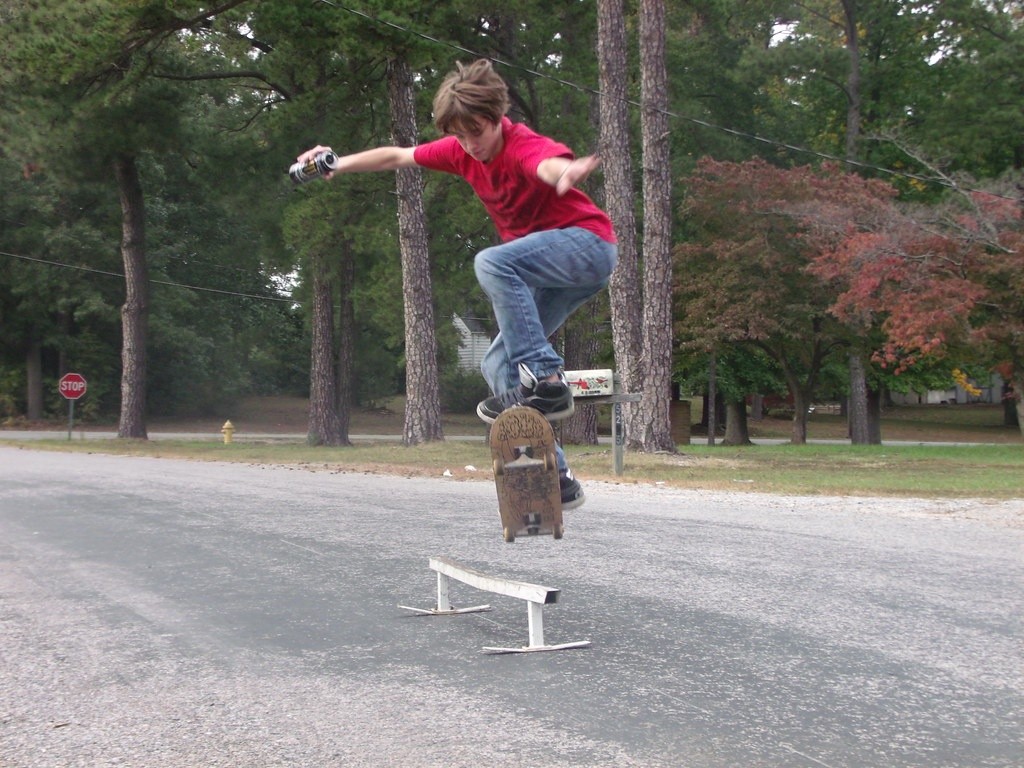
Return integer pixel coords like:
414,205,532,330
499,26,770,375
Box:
59,372,86,400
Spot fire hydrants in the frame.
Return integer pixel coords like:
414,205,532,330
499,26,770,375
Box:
221,419,236,444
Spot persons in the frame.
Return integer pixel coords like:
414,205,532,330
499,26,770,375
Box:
294,57,618,515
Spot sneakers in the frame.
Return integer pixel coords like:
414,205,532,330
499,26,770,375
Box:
558,467,585,510
476,362,575,426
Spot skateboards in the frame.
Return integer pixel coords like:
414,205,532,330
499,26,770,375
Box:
489,406,565,543
